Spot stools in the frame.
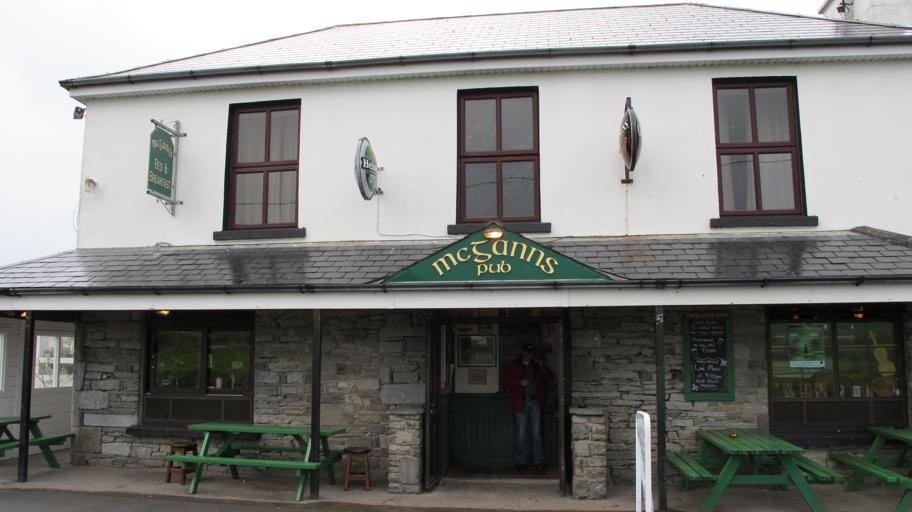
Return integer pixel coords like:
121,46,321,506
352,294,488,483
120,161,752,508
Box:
166,442,198,486
342,447,372,491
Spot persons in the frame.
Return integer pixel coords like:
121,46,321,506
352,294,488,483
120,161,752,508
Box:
505,343,555,475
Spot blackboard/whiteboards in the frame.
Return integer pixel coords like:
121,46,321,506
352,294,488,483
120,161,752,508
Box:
682,305,735,401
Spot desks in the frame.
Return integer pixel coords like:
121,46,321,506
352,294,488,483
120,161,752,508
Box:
847,426,912,512
680,429,826,512
0,413,60,468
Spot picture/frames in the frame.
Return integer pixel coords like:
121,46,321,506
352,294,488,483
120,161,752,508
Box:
457,335,497,368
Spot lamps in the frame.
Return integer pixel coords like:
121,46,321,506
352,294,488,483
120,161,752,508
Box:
484,228,505,239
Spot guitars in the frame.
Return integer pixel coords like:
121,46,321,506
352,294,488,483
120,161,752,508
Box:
868,328,898,378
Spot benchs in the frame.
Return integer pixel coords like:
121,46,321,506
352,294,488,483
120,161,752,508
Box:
831,452,912,487
793,455,845,483
0,432,76,458
219,445,347,456
165,454,324,471
665,451,713,482
